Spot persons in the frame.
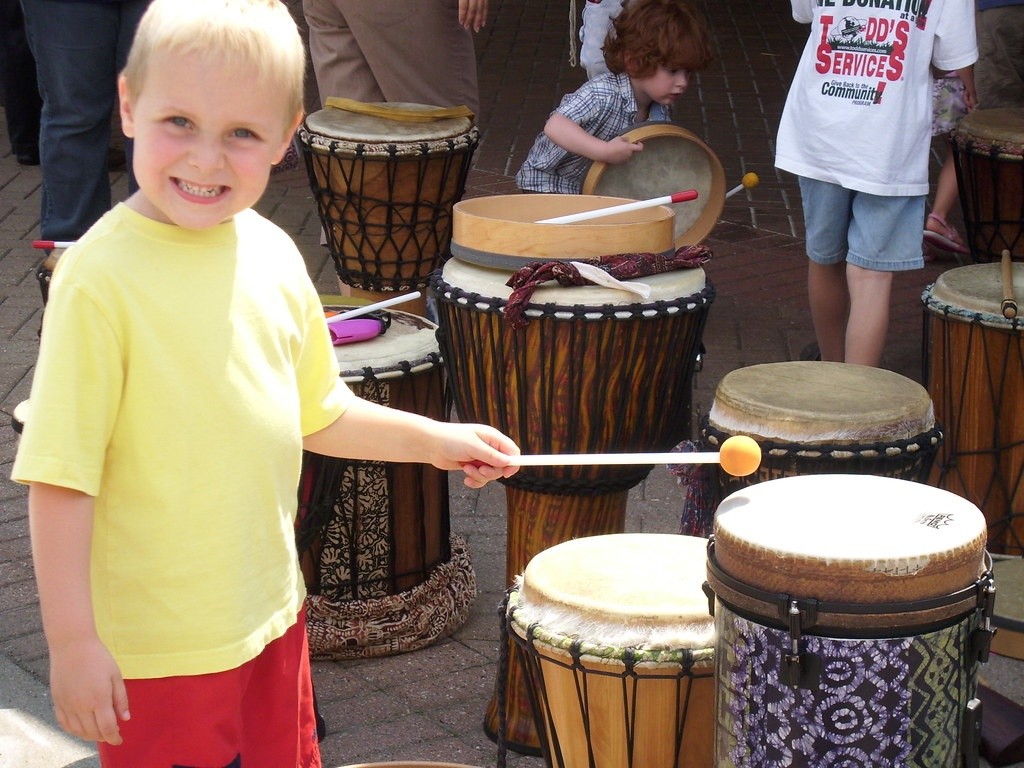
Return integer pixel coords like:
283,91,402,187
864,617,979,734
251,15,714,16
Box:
10,0,520,768
0,0,1024,372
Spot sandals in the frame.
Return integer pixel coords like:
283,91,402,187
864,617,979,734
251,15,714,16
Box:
920,213,970,255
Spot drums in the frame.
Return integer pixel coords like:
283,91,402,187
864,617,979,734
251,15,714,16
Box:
579,121,728,257
918,260,1024,562
989,558,1024,662
294,101,480,320
497,467,1000,768
695,360,945,542
11,240,68,463
424,191,717,755
948,104,1024,265
294,296,478,660
